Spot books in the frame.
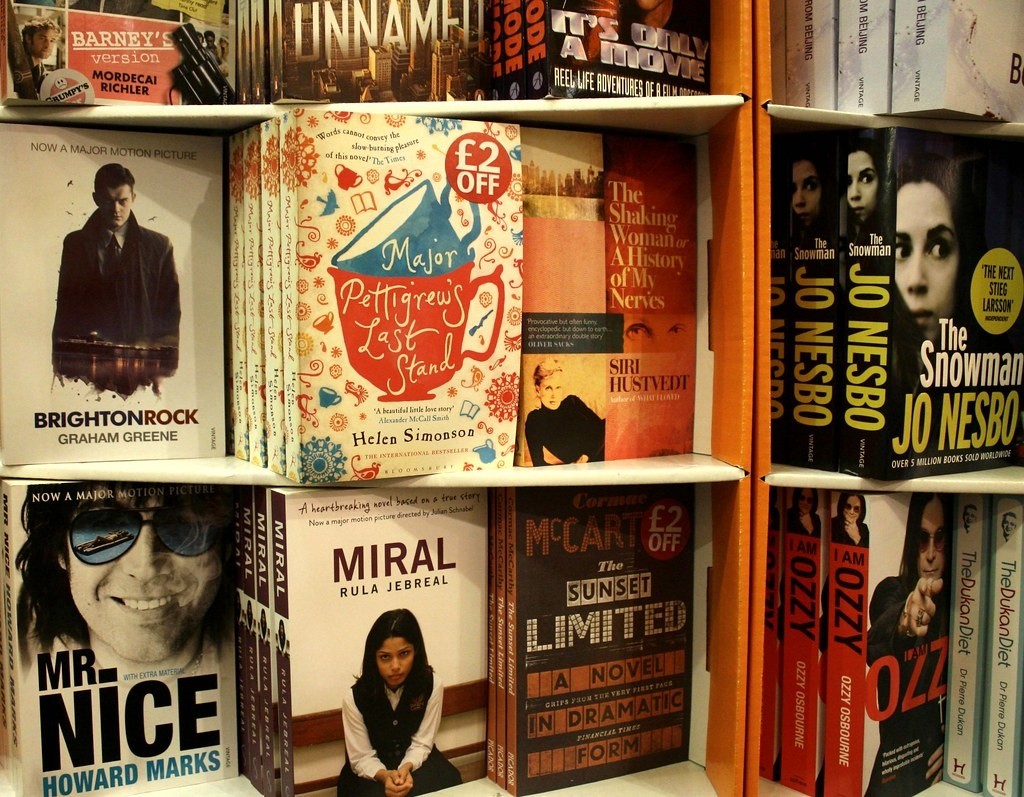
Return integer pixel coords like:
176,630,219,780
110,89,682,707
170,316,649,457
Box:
1,0,1023,121
1,479,1024,797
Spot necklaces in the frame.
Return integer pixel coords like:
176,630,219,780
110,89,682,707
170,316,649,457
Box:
190,655,202,673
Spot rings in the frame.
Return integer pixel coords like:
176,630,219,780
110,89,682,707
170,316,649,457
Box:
917,610,922,626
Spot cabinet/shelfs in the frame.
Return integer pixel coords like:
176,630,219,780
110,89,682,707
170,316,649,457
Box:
0,0,1024,797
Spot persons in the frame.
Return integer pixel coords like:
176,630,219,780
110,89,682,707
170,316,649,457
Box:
336,608,462,797
787,487,821,538
218,35,229,75
843,137,883,236
831,493,869,546
524,358,604,465
769,488,779,529
0,108,1023,480
893,149,960,391
204,30,217,55
19,485,236,679
867,492,950,797
21,15,59,87
53,163,181,395
792,153,823,233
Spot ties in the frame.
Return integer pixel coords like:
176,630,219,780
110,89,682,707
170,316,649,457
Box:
105,233,120,277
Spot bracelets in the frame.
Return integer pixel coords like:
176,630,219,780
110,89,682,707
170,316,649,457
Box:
904,591,915,637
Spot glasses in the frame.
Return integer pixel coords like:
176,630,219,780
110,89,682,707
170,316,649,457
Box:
64,493,229,565
919,530,947,552
801,495,813,502
845,504,860,512
